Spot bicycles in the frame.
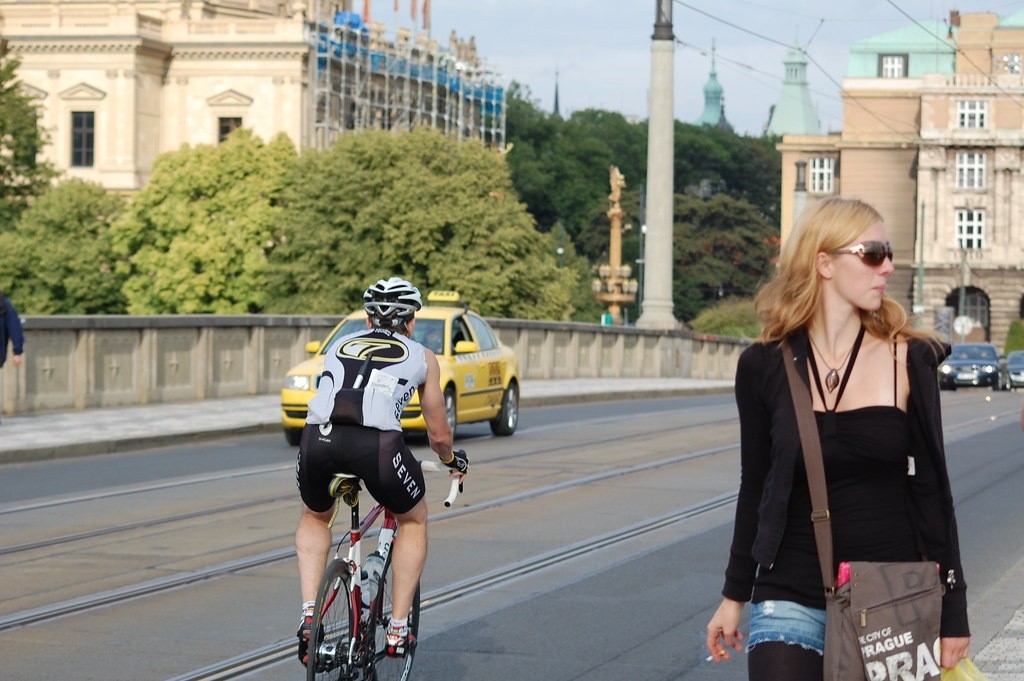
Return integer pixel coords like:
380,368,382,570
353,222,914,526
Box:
304,450,468,681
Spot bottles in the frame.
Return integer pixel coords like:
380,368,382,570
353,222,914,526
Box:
606,312,612,327
601,313,605,326
363,551,385,599
361,566,370,620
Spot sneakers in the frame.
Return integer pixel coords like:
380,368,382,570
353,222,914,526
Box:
297,606,324,668
385,623,417,658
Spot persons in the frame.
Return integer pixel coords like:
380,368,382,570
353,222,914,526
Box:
959,348,990,359
295,276,470,673
706,196,971,681
0,293,24,367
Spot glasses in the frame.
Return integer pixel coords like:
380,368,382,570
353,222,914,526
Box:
831,240,893,266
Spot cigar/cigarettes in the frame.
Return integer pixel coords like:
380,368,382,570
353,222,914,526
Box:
706,649,726,663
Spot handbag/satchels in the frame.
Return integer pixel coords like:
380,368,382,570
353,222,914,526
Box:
822,560,942,681
941,657,987,681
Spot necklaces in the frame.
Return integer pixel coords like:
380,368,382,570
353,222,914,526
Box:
810,335,854,394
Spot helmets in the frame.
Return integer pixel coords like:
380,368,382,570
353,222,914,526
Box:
363,277,422,327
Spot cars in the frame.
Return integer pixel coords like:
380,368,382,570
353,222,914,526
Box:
278,290,523,439
936,343,1009,391
1005,350,1024,387
